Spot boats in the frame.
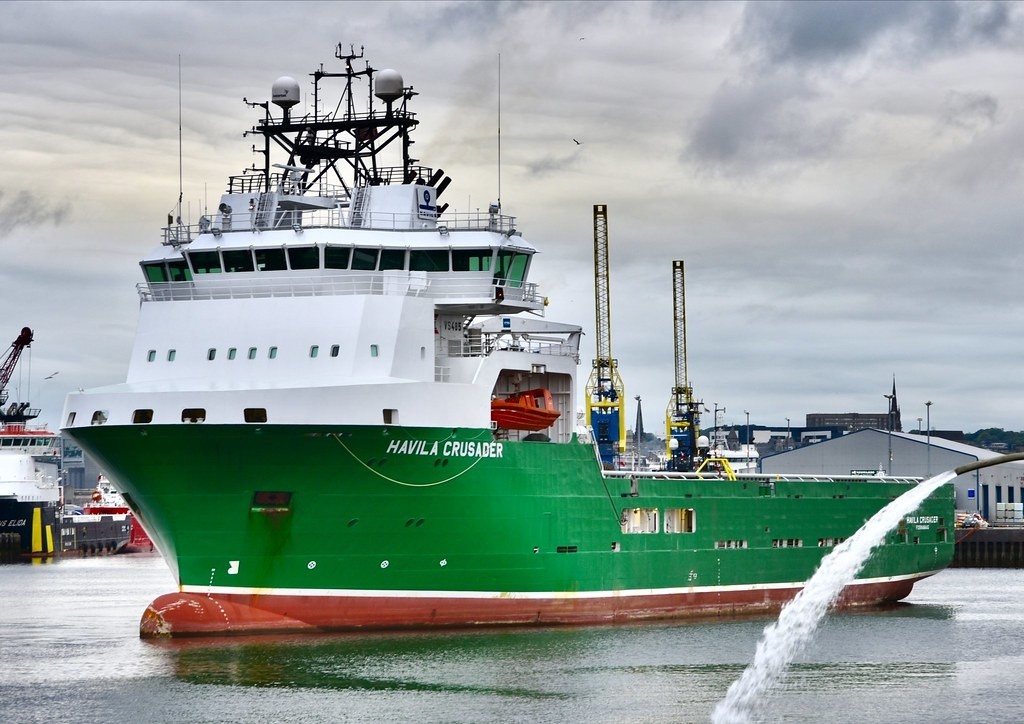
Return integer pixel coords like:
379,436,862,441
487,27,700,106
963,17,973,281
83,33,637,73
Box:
491,390,560,432
0,401,133,564
707,435,759,471
59,41,958,640
82,472,157,551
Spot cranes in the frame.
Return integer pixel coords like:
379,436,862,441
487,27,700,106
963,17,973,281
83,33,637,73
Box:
1,327,35,405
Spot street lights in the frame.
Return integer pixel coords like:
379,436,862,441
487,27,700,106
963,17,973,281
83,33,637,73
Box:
696,435,710,463
883,394,894,476
924,400,933,479
743,409,750,473
668,438,678,471
785,417,790,451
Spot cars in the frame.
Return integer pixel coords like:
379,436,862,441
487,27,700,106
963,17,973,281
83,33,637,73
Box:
962,517,978,528
